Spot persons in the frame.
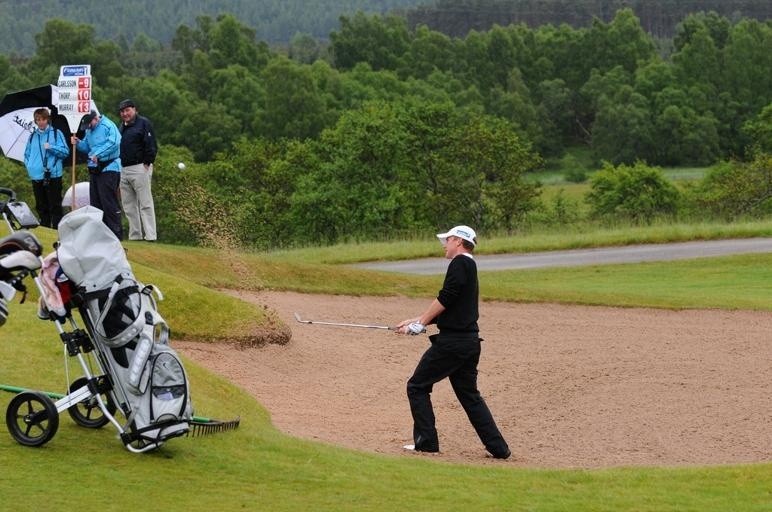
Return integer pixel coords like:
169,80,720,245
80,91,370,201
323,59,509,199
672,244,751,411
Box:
393,223,512,459
70,110,122,238
114,99,158,241
23,108,69,228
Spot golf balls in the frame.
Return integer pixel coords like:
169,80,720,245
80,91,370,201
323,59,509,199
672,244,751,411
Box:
178,163,185,169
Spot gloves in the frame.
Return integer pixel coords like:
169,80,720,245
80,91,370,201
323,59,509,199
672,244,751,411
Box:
405,321,425,335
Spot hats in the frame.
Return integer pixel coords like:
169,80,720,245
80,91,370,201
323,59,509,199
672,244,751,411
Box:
437,224,477,247
120,99,135,110
80,110,96,130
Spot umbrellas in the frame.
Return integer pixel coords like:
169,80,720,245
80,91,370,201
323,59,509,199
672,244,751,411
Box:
0,84,99,170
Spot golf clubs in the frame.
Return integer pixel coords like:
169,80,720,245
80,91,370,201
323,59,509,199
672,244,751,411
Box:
0,230,45,326
294,312,426,334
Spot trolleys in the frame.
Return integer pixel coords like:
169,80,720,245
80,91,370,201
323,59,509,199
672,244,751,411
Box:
0,188,194,456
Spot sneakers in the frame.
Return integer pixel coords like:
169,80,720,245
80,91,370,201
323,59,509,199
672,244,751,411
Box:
404,444,439,455
